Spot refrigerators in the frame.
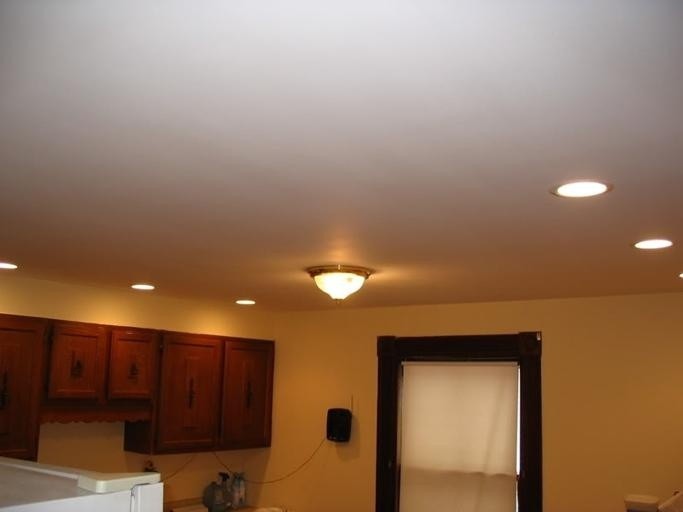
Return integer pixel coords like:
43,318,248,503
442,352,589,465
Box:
0,454,162,511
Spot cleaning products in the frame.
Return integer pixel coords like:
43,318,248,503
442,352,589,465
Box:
219,471,233,512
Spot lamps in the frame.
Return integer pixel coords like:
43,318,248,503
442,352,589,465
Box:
306,265,371,305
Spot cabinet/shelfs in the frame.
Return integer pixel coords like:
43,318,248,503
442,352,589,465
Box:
40,317,159,423
0,313,49,461
124,331,275,456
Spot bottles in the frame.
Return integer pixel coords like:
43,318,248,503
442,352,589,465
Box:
231,472,246,509
204,482,224,512
